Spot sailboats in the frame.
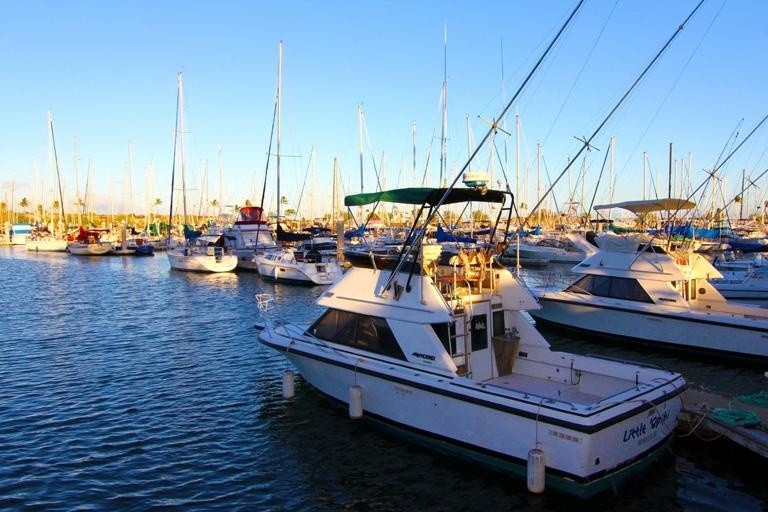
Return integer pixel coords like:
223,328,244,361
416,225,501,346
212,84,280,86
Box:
253,0,704,496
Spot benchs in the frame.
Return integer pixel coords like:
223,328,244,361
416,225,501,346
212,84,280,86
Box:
437,255,493,297
